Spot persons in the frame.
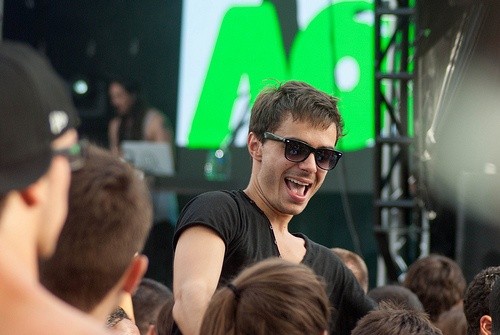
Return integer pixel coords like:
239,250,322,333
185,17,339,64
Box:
0,40,500,335
104,68,175,159
172,80,379,335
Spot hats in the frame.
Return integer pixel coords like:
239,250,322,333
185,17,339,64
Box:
0,39,81,192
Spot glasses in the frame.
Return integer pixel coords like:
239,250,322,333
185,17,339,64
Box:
262,129,342,172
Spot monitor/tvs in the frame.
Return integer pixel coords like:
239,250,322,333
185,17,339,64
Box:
122,141,174,177
